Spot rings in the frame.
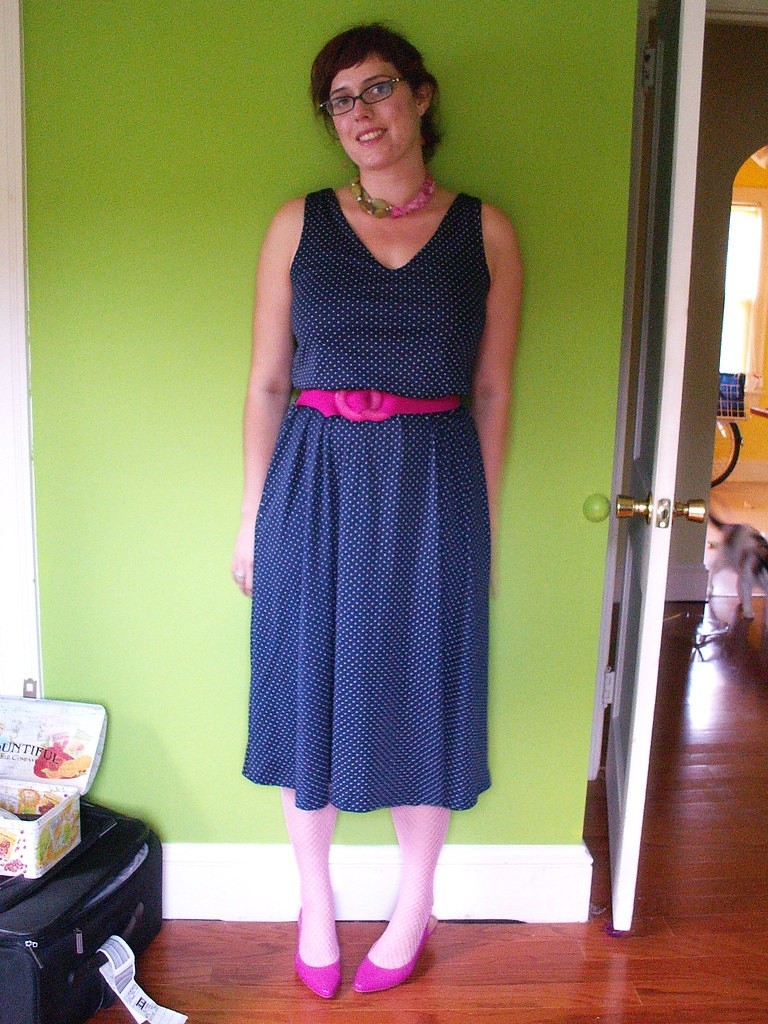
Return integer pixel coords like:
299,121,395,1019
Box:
235,571,244,579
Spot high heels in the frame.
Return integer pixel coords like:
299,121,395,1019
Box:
295,909,342,998
353,916,439,993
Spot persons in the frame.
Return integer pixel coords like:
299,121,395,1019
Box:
231,24,524,999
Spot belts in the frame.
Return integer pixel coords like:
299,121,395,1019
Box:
297,389,460,422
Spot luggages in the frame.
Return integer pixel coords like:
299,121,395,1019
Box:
0,800,162,1024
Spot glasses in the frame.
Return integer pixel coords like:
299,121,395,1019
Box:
317,77,406,116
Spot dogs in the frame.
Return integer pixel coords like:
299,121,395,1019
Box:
706,512,768,620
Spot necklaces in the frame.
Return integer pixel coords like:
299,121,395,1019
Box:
352,172,435,219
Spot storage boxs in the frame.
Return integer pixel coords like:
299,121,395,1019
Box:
0,695,109,879
